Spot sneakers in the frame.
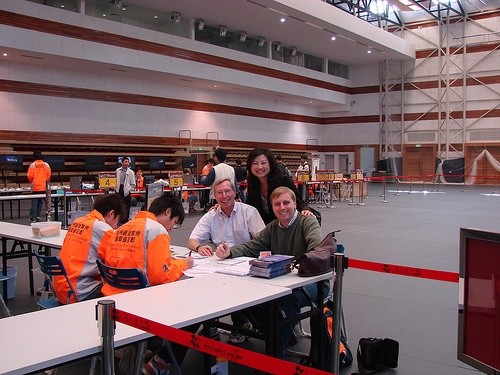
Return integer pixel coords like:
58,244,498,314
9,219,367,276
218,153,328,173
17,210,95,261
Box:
28,217,41,222
140,356,170,375
118,346,136,375
207,327,221,338
228,321,258,342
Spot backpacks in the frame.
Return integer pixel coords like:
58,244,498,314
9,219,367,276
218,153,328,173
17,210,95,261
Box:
309,299,354,371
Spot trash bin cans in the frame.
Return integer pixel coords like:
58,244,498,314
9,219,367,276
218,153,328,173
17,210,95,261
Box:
0,265,17,299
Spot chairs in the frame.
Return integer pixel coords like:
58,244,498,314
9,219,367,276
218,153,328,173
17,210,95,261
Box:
94,258,184,375
31,249,80,310
281,244,349,346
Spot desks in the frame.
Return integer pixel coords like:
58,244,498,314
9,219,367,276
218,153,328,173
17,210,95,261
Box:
0,179,334,375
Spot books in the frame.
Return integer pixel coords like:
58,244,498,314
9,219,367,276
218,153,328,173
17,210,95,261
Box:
248,255,294,280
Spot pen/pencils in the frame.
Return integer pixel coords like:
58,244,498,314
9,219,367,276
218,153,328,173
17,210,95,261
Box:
189,251,193,257
224,241,226,257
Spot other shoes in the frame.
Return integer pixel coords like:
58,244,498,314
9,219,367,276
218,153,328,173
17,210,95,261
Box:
201,208,205,211
288,330,298,346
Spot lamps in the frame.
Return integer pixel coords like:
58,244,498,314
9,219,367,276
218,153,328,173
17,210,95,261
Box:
219,25,228,37
239,31,247,42
196,18,205,30
257,37,265,47
290,46,298,56
170,12,181,24
275,41,282,51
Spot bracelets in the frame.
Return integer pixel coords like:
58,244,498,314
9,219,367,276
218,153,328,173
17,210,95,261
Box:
196,244,203,253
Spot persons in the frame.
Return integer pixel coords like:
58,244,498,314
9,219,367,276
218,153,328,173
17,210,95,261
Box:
187,163,267,344
95,194,193,375
114,157,136,225
131,149,309,204
216,188,330,360
244,148,314,226
52,195,128,304
27,151,51,222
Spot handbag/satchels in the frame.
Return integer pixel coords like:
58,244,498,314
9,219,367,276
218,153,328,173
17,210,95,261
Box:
292,230,341,277
351,338,399,375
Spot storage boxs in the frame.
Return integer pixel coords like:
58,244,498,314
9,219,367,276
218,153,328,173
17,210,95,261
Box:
31,221,62,237
0,265,18,300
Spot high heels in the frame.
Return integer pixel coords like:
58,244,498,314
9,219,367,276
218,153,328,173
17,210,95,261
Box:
189,207,197,213
